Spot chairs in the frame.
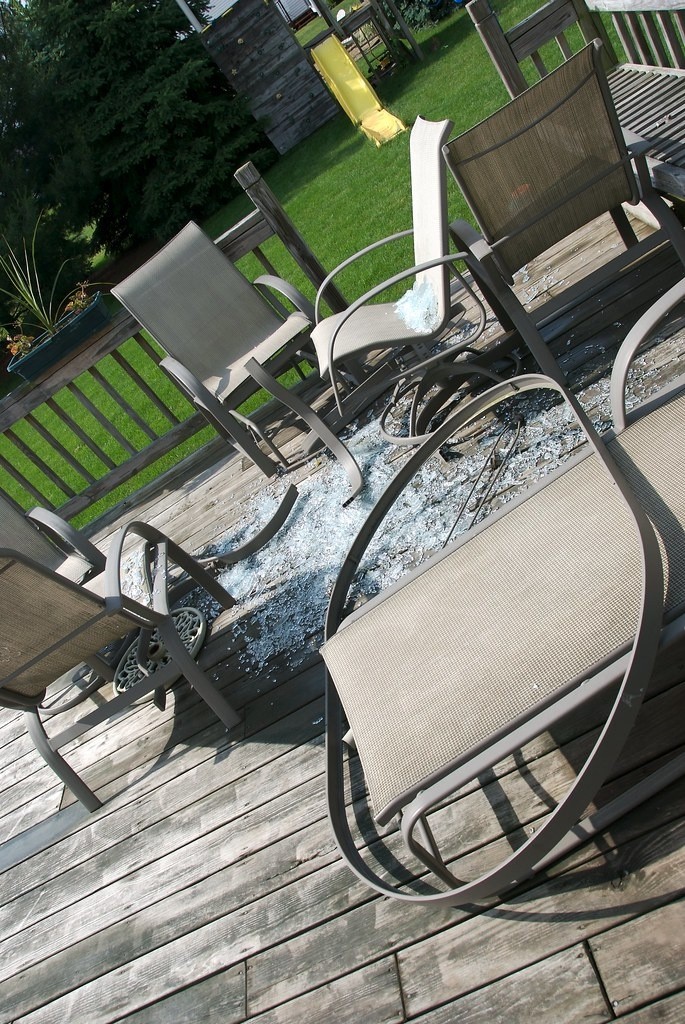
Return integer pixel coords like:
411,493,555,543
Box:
465,0,685,231
315,274,685,902
0,521,241,815
309,115,522,444
0,492,140,715
446,38,685,384
108,221,365,479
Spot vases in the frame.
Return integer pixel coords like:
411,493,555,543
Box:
6,291,112,381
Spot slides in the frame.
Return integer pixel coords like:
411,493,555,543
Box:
311,34,405,150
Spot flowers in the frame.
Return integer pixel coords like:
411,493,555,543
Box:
0,209,116,355
